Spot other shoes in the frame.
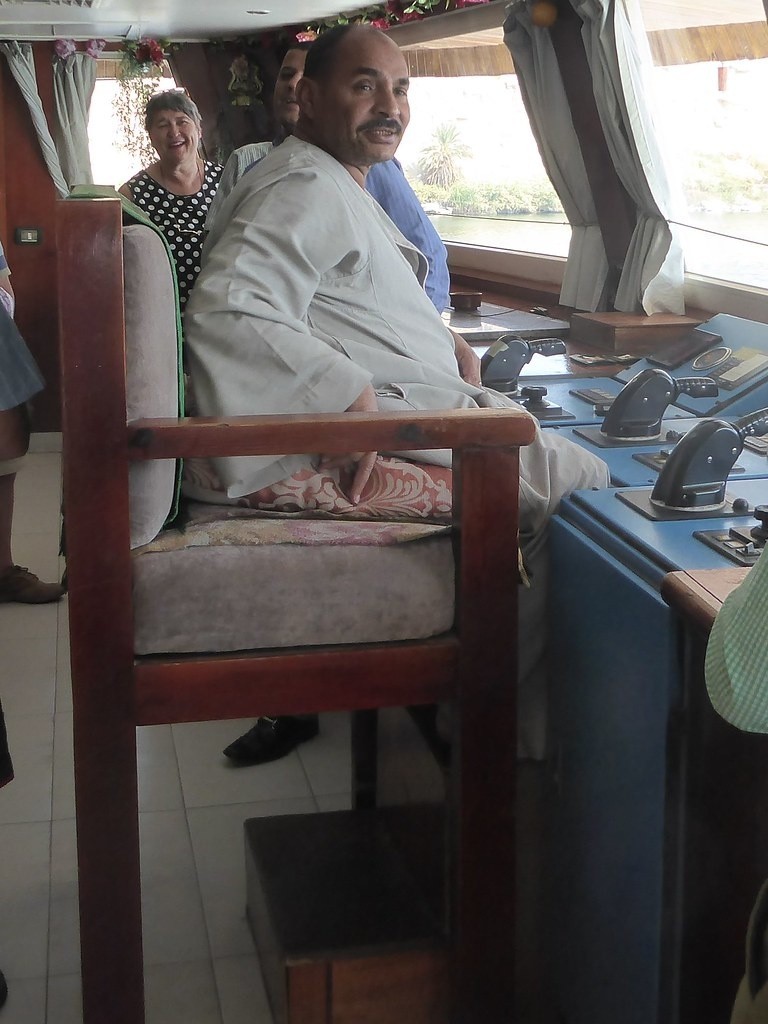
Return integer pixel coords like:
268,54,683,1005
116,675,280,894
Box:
223,714,320,766
0,565,64,605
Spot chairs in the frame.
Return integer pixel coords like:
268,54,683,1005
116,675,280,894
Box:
51,187,542,1024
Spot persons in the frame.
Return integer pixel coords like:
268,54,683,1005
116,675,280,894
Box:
117,91,225,374
1,238,65,606
184,21,608,766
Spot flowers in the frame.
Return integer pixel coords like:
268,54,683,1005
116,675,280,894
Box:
84,38,108,59
296,0,488,47
54,35,80,60
112,35,170,169
